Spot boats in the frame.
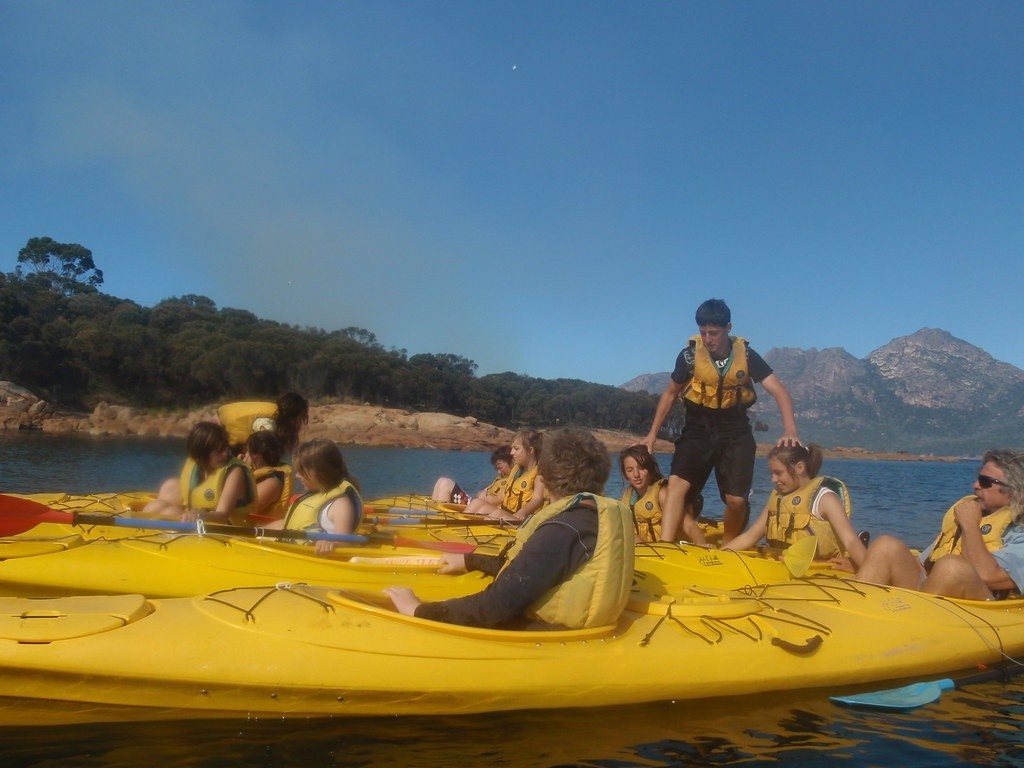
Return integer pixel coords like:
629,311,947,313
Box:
0,489,1024,726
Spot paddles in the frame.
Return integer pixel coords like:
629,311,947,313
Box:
829,662,1023,710
363,507,443,516
0,494,479,553
362,517,522,526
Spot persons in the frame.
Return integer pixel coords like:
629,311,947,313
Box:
162,421,258,523
431,428,550,520
630,299,804,547
213,394,309,444
145,430,294,518
262,437,362,555
720,439,868,573
621,444,716,549
382,425,634,632
829,448,1024,600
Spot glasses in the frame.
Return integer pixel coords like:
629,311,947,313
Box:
977,473,1009,489
